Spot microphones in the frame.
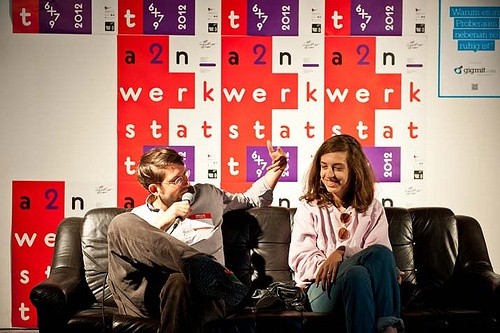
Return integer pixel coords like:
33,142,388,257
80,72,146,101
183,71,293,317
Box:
173,192,194,229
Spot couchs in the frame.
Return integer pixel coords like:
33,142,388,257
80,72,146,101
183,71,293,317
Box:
29,206,500,333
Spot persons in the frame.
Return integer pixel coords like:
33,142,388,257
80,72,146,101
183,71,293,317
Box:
106,140,288,332
287,133,407,333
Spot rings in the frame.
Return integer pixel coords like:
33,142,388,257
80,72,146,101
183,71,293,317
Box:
327,270,330,273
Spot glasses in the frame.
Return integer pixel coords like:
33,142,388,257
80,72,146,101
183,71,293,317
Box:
156,168,191,186
338,211,352,242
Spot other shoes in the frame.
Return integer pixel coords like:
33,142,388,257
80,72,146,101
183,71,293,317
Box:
189,254,249,306
